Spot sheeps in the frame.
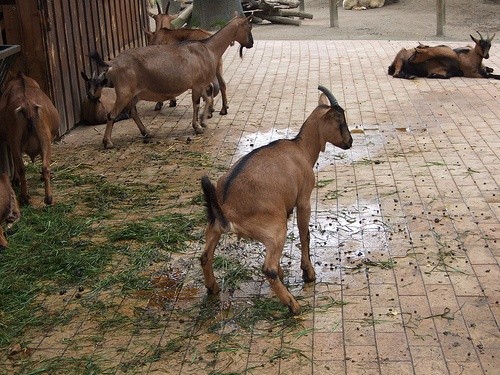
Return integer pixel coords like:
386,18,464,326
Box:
0,71,61,250
198,85,354,316
80,1,254,151
387,29,500,80
